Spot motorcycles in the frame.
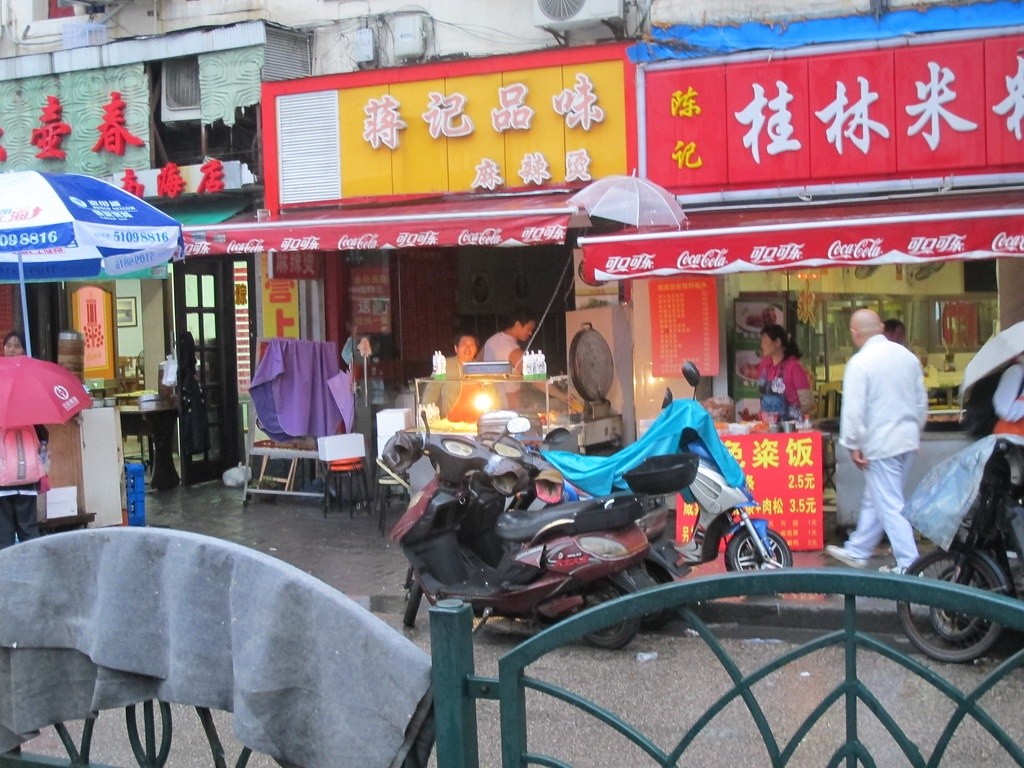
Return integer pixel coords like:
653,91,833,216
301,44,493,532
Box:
505,360,794,574
380,429,652,652
897,434,1024,662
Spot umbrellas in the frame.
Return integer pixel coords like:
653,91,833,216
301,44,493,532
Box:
567,167,688,229
0,171,186,358
0,356,92,425
959,320,1024,438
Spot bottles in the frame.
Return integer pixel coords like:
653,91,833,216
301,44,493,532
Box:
39,440,48,464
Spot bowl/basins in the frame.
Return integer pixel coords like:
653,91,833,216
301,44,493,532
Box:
92,397,117,408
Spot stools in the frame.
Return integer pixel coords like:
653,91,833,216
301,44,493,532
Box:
323,456,410,538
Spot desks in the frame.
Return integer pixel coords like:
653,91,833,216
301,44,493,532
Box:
119,405,180,491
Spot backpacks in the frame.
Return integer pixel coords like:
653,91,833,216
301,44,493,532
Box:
0,427,46,487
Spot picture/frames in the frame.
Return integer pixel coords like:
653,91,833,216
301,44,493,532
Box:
116,297,138,328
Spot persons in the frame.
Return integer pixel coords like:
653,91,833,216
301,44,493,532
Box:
991,352,1024,436
2,331,24,357
423,334,501,425
476,311,577,408
0,424,48,552
740,324,817,422
826,308,928,585
882,318,905,345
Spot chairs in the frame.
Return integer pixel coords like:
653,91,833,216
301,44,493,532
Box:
116,365,145,394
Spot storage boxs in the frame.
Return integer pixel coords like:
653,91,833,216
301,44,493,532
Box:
477,408,543,448
376,407,413,460
318,432,366,461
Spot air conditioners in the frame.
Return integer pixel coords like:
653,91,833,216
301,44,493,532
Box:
534,0,623,33
160,25,310,122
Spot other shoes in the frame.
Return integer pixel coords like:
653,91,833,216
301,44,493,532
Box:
878,565,923,577
826,545,866,569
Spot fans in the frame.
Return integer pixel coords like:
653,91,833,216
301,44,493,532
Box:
138,350,144,374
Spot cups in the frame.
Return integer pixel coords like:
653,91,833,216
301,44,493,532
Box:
769,420,796,432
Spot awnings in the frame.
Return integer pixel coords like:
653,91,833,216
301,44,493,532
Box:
155,199,250,225
576,190,1024,283
182,193,582,256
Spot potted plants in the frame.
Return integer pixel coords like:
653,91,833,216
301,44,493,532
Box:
249,476,280,503
